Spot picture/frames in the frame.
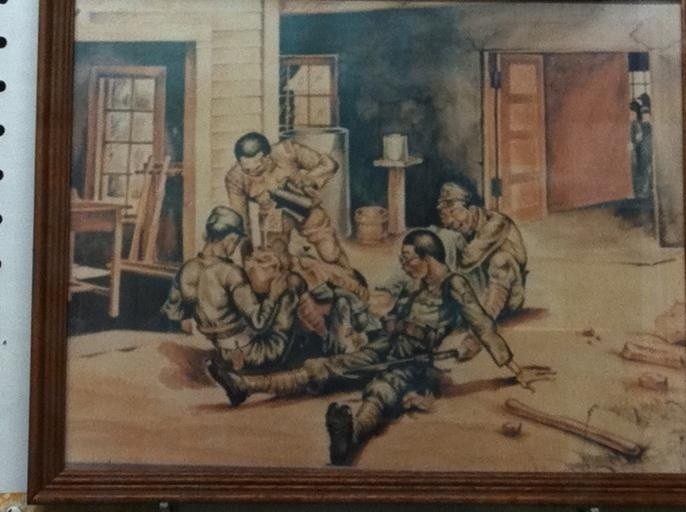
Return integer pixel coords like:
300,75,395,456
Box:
26,1,686,508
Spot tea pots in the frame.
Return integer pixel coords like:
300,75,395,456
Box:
267,176,317,224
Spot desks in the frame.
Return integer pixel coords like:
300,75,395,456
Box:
70,199,133,318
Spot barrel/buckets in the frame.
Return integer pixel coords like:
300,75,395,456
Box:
355,206,390,242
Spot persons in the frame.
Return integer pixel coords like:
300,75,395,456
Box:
221,132,371,300
366,179,533,364
157,207,370,371
631,94,658,202
205,226,556,465
242,243,393,328
